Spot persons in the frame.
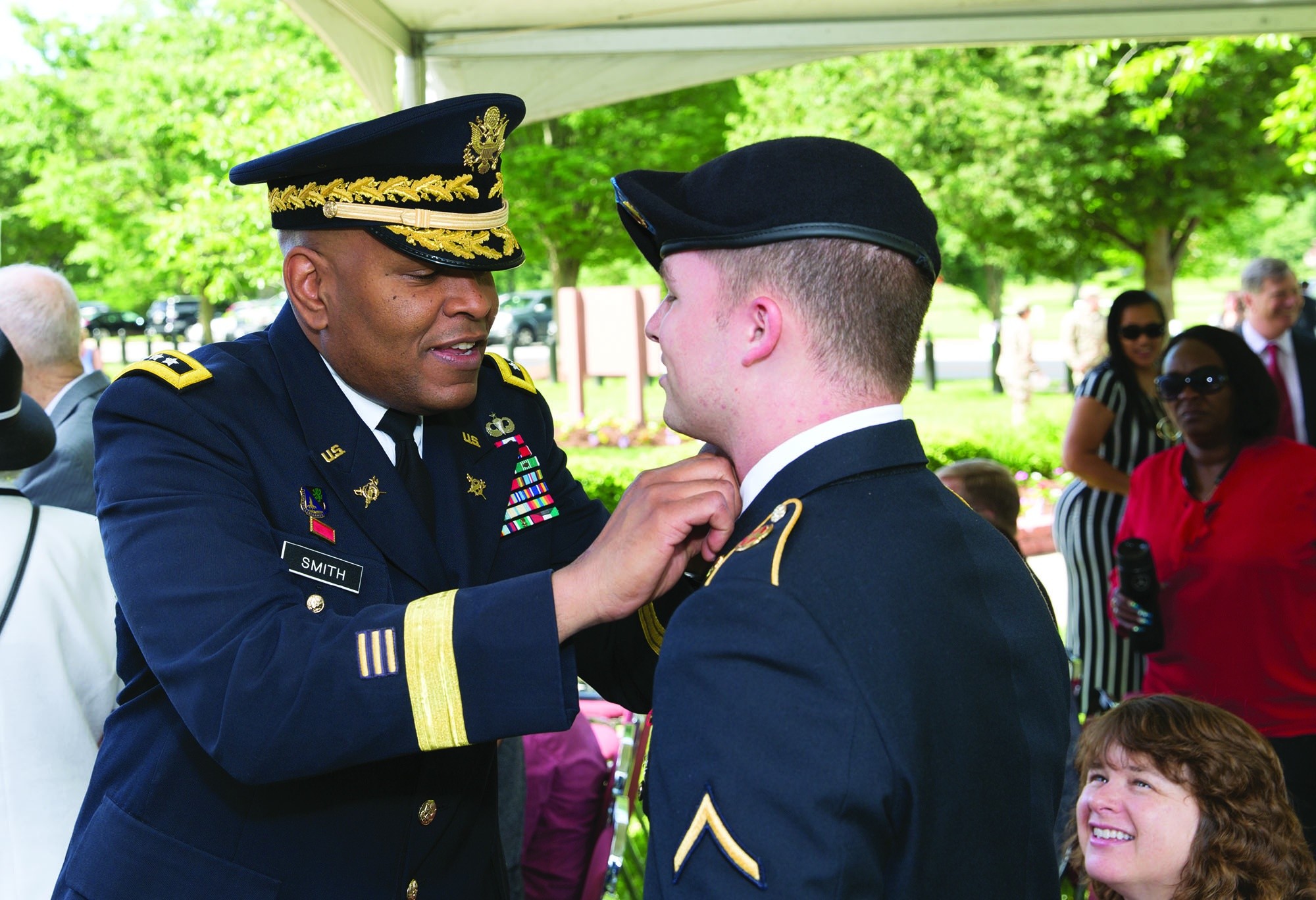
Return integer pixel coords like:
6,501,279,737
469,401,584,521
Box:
1066,695,1316,900
1231,257,1315,447
521,703,619,897
933,454,1057,619
1107,324,1316,862
610,135,1074,900
0,262,114,514
0,330,119,900
50,92,741,900
1052,290,1182,723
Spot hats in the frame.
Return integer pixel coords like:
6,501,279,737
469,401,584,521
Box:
610,136,942,283
230,93,526,271
0,327,57,472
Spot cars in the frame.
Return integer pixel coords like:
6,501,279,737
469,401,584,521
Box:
484,290,557,347
81,306,148,338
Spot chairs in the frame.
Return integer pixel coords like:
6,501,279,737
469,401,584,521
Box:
581,795,630,900
595,722,637,834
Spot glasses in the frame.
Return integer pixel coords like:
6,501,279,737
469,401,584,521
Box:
1153,365,1235,403
1121,321,1165,341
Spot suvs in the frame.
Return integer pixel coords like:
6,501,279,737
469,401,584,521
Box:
147,296,225,338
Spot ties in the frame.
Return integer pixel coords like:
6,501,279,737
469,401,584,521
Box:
1266,344,1298,442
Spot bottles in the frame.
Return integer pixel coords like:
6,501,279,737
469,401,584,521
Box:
1117,538,1165,654
